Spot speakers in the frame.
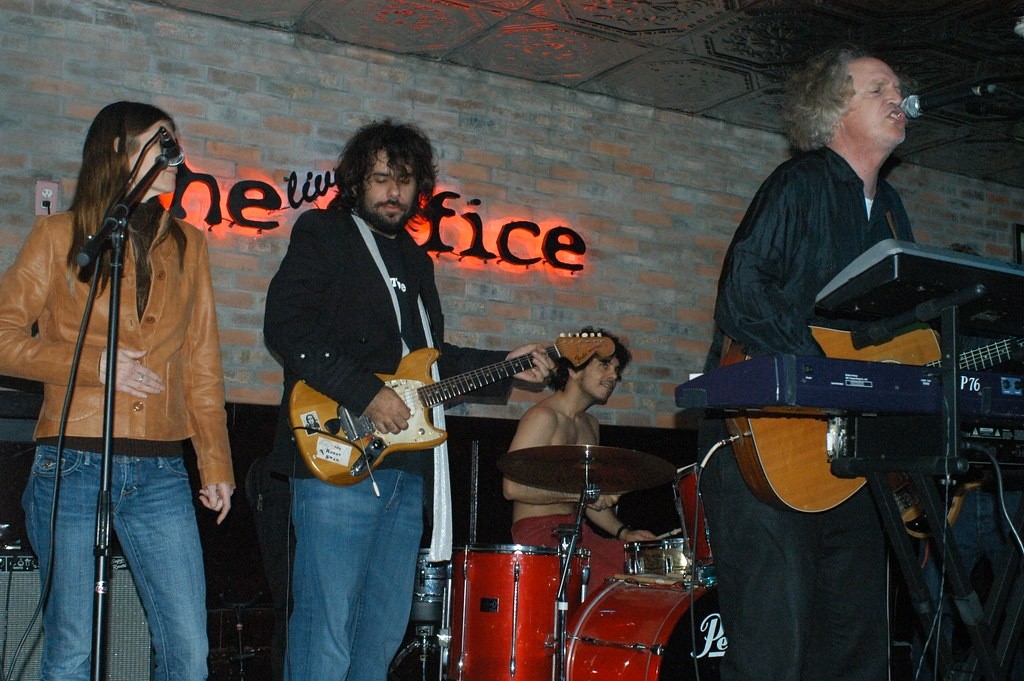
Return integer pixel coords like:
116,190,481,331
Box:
0,541,155,681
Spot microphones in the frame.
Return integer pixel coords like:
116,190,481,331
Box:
898,85,995,119
157,126,185,167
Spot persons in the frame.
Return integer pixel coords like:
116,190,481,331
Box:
698,39,1024,681
305,414,321,436
0,101,236,681
503,326,657,629
263,118,555,681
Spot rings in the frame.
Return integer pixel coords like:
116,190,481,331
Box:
139,374,144,381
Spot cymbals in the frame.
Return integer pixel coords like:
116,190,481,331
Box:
497,444,677,496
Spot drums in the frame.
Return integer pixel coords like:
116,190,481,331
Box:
562,576,729,681
672,462,715,572
387,641,447,681
448,546,592,681
411,547,453,623
623,538,690,576
207,606,282,655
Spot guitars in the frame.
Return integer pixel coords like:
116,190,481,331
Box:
287,334,616,488
724,302,1024,517
884,469,983,539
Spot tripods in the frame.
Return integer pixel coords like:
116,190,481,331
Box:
387,638,449,681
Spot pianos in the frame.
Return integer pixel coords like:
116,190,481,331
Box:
814,239,1024,340
675,354,1023,423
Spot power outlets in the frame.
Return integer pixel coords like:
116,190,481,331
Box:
35,180,60,216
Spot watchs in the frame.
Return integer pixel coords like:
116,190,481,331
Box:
616,520,632,539
